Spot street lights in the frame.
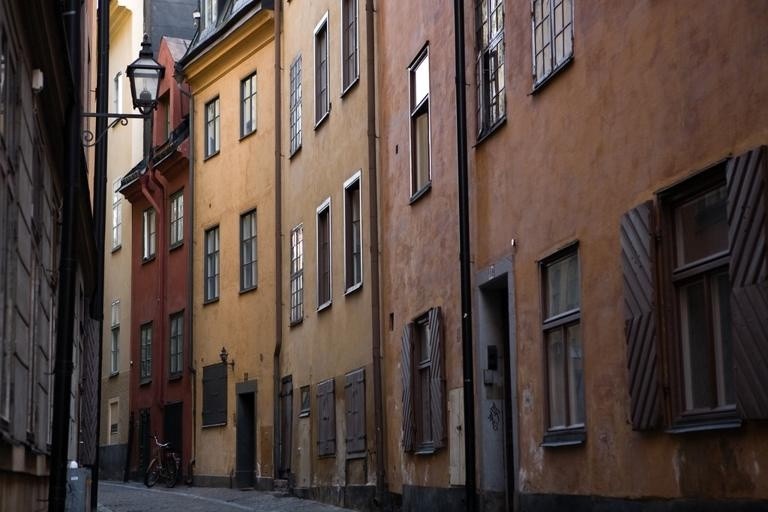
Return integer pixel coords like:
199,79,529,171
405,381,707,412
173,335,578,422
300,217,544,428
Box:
41,29,167,512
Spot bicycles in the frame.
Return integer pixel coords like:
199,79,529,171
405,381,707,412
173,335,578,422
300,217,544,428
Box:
144,436,181,489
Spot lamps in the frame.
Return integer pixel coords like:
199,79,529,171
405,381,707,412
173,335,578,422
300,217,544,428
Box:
74,33,166,147
177,11,201,59
220,346,234,372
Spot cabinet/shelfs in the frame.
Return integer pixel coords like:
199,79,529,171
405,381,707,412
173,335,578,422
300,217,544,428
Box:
32,68,44,113
